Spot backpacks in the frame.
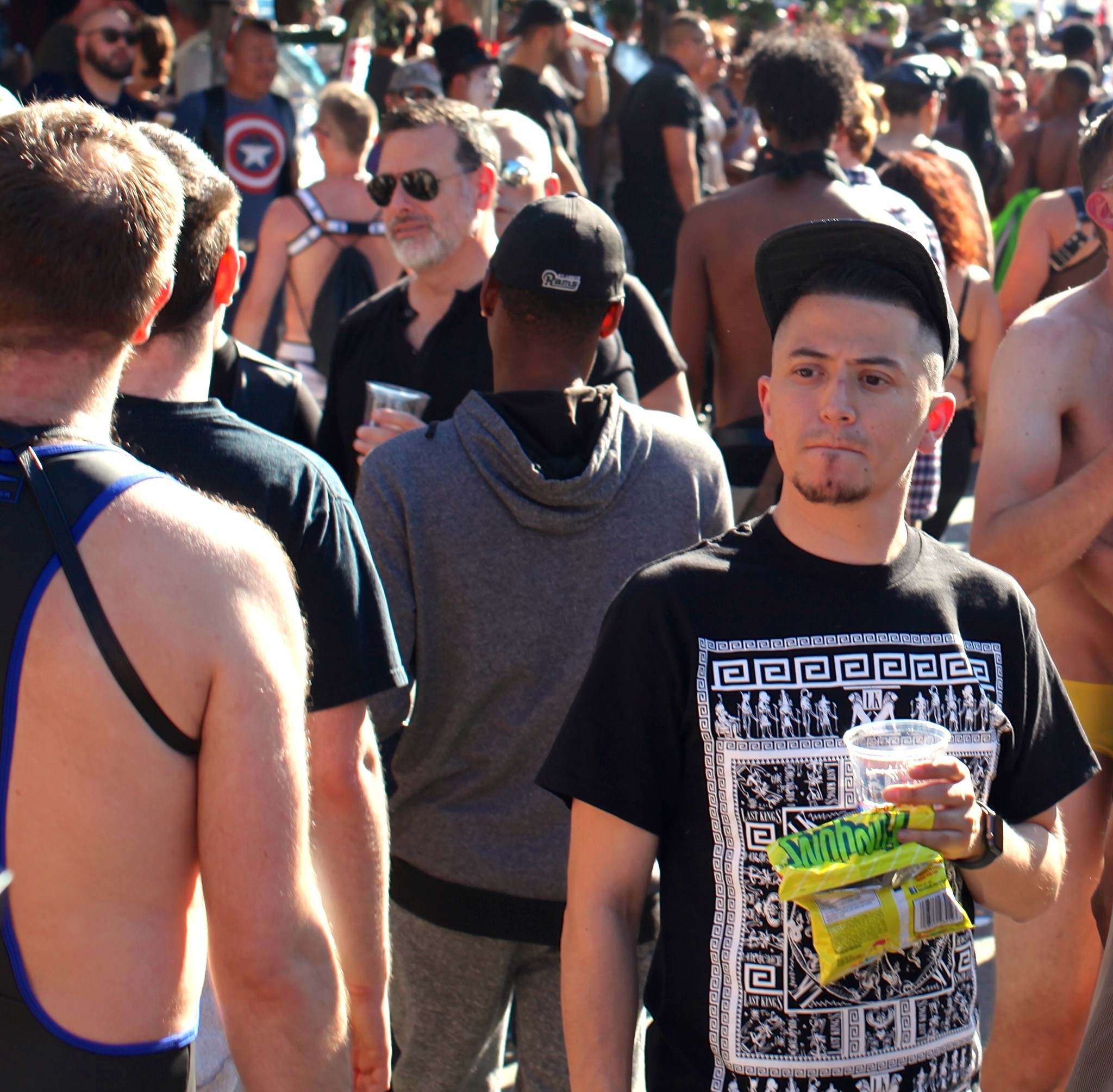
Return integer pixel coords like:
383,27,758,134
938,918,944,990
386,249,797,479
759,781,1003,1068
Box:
281,195,383,376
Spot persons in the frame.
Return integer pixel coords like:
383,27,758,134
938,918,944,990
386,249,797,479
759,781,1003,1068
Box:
1,0,1113,1092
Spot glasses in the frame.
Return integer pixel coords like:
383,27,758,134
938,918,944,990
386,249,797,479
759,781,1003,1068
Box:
86,29,136,44
498,161,529,188
387,89,426,100
366,168,476,206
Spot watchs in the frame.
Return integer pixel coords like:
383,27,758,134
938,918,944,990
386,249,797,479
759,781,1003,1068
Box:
949,801,1005,869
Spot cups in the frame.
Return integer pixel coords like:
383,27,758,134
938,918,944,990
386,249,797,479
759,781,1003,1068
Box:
364,381,430,428
844,719,951,809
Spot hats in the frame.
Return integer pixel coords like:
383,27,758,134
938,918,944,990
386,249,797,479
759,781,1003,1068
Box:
899,51,951,88
754,219,960,380
389,64,442,98
433,25,498,89
76,0,139,27
491,192,626,303
506,0,571,35
